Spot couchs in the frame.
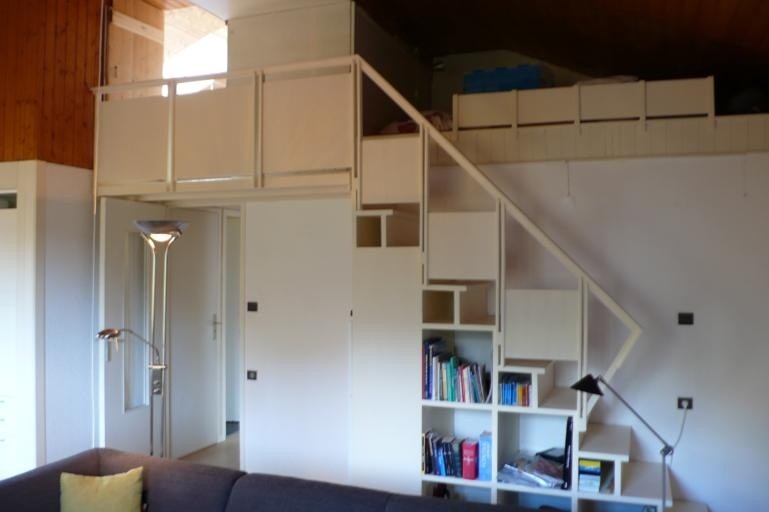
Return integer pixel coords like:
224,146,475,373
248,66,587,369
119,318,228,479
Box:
1,449,534,512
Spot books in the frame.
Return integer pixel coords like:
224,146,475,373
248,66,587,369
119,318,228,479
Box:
500,375,532,405
422,427,492,480
497,416,573,489
578,459,601,494
423,337,492,404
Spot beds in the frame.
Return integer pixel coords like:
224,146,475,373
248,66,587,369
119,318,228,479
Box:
379,75,768,161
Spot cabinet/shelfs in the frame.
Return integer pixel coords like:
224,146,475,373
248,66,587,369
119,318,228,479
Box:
357,205,673,510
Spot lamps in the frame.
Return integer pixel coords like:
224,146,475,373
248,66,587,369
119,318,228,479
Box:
98,215,188,461
571,372,675,510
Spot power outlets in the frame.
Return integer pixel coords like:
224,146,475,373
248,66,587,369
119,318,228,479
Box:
678,395,694,411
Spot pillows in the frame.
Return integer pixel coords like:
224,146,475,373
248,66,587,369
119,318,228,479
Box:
58,466,147,511
